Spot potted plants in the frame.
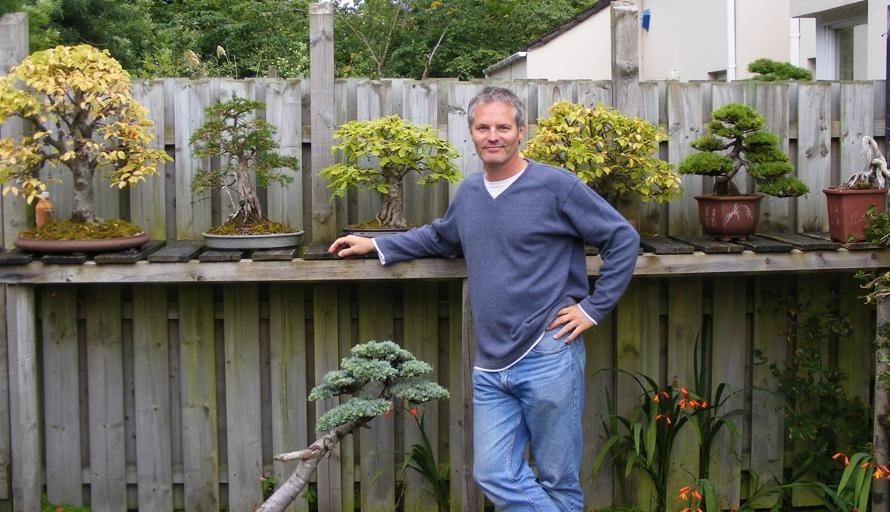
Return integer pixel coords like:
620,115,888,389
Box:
0,44,462,253
520,100,889,245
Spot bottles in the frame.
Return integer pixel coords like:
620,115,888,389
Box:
35,191,54,228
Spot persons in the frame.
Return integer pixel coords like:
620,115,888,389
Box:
326,85,643,512
